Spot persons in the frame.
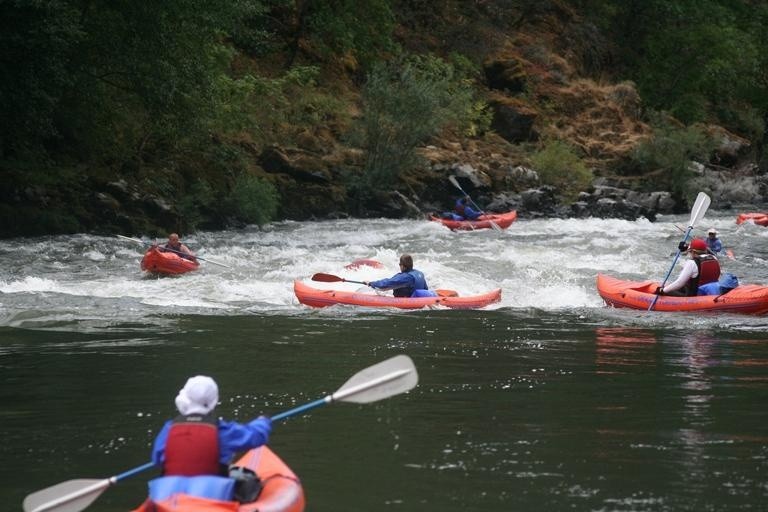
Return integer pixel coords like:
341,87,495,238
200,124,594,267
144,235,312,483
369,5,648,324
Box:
153,233,196,259
694,229,721,252
363,255,428,296
656,240,720,297
456,196,485,220
152,376,273,476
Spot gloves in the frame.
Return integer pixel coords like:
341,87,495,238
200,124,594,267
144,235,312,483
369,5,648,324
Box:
656,286,664,294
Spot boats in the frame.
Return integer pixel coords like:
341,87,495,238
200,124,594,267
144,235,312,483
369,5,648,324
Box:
735,212,767,226
427,209,518,231
293,271,504,315
131,445,305,512
344,259,384,272
595,271,768,315
138,244,200,280
676,246,738,264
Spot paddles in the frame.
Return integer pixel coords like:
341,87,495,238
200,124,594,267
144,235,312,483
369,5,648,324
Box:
24,354,417,512
449,176,502,232
649,191,711,310
116,235,230,269
312,273,366,284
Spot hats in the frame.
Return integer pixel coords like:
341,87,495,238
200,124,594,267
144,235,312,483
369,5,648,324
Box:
176,375,219,416
706,227,717,234
688,239,707,250
